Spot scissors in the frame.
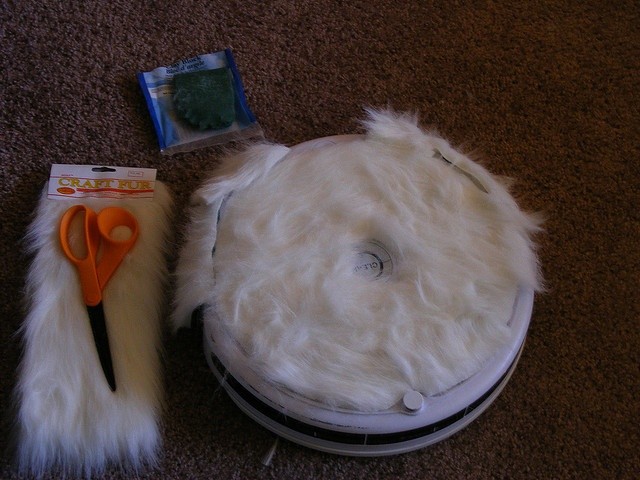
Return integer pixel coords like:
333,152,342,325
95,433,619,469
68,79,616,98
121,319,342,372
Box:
59,204,140,392
